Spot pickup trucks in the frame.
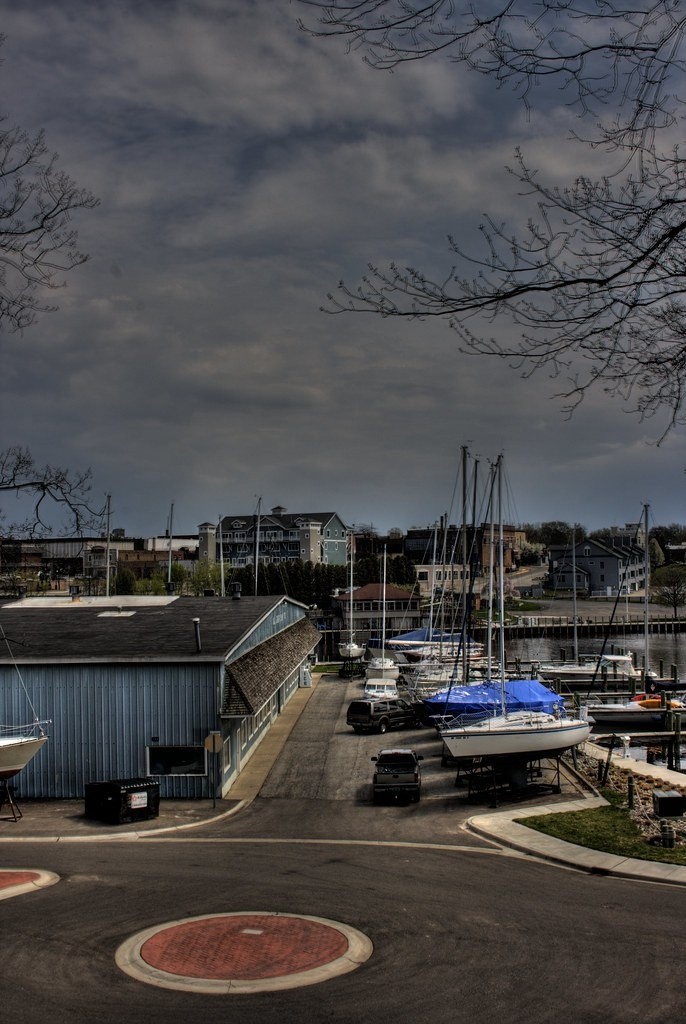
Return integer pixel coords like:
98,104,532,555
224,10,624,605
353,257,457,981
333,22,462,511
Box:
371,748,425,803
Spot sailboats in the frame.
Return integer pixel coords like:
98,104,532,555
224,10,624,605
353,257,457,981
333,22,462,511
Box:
337,443,686,760
0,624,53,779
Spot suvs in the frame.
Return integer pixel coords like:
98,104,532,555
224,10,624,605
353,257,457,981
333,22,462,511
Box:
347,696,422,734
